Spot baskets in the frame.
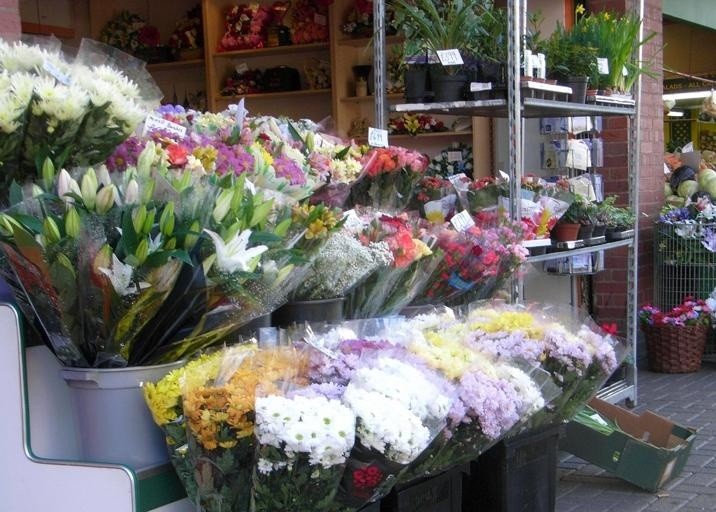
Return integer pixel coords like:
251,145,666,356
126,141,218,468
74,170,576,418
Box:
642,320,708,375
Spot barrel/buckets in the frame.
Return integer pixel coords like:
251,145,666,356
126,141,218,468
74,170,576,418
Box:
57,361,183,468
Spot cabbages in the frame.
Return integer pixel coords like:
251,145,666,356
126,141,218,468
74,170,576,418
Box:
664,165,716,210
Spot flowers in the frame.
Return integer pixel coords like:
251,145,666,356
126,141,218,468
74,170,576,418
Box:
640,295,712,332
0,0,620,509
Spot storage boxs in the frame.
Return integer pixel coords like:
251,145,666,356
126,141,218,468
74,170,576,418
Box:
556,395,696,493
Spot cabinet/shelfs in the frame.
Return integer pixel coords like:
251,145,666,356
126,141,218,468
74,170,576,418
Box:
364,0,643,408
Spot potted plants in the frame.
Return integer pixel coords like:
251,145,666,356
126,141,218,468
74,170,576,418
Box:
612,9,667,100
605,207,636,239
